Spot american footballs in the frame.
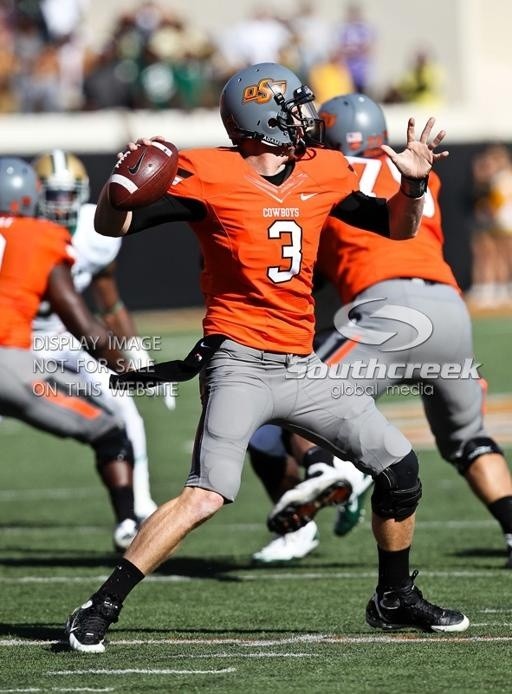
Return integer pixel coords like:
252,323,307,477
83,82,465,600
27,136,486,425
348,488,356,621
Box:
107,139,178,208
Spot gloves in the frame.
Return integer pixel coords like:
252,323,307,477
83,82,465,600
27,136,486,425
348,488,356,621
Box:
65,203,123,294
117,335,177,412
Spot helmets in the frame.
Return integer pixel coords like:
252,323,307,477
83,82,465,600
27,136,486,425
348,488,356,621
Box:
0,156,44,218
28,148,90,227
219,62,327,159
317,92,390,159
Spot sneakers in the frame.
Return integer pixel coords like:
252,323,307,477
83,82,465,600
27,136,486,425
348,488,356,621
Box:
266,456,353,535
112,518,143,554
63,592,124,654
252,519,321,562
333,473,376,538
502,533,512,569
133,498,158,521
365,569,470,632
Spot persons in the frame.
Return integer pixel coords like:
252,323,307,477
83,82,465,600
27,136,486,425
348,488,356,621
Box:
472,144,512,309
1,0,373,109
266,95,511,569
251,267,372,564
31,149,174,523
0,157,140,555
66,63,470,654
